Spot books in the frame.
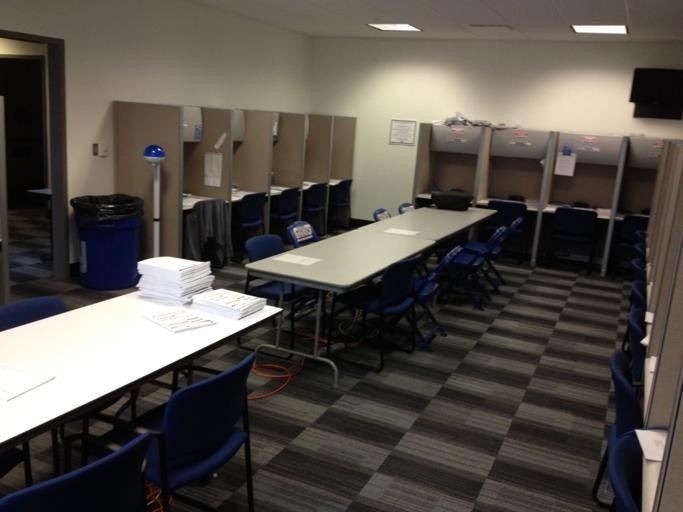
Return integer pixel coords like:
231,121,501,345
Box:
135,256,215,305
142,307,217,332
190,288,267,320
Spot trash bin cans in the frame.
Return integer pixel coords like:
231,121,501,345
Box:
70,193,145,289
